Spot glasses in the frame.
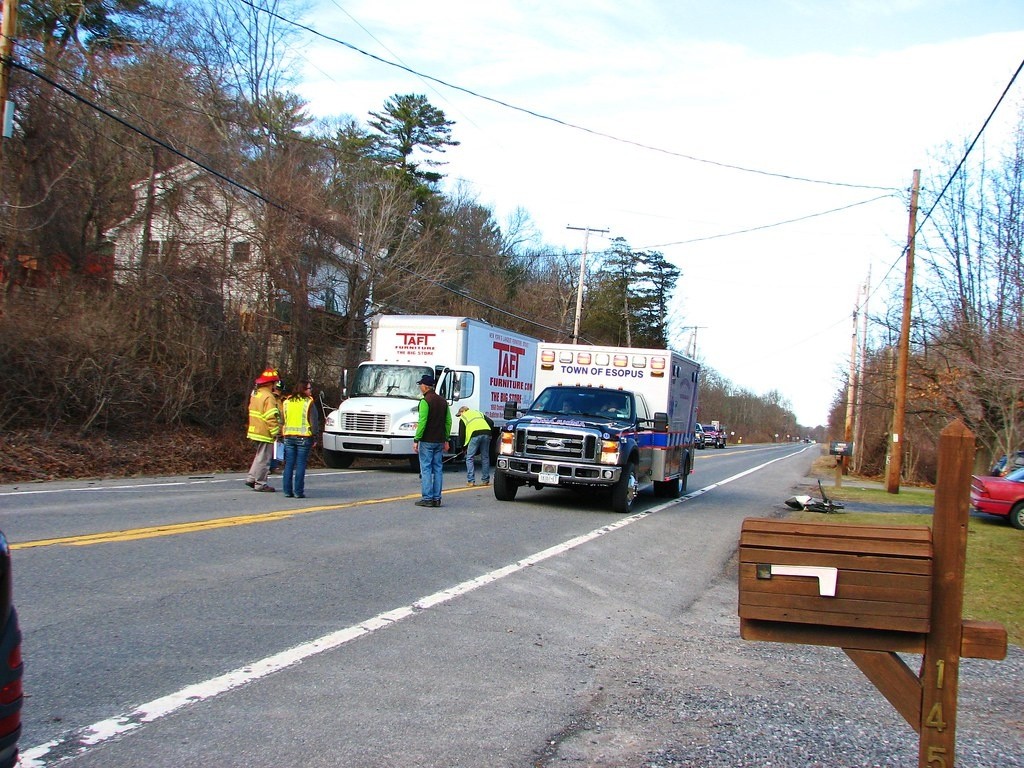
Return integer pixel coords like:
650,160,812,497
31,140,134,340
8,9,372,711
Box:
307,387,313,390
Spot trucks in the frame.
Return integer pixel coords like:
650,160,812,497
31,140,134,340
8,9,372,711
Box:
493,342,701,514
318,313,545,469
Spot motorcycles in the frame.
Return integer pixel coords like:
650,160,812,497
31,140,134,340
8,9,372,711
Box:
709,429,728,449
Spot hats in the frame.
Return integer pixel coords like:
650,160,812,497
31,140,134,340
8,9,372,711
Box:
254,368,279,384
417,375,437,386
455,406,469,417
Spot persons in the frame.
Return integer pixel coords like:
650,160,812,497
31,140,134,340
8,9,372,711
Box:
279,379,319,498
558,398,575,412
456,406,494,486
245,368,281,492
600,397,629,414
413,375,452,507
267,379,287,475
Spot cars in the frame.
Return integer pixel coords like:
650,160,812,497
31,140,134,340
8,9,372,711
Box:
0,531,24,768
694,423,705,450
989,450,1024,477
970,465,1024,530
811,440,816,444
804,437,811,443
799,440,804,443
702,425,718,445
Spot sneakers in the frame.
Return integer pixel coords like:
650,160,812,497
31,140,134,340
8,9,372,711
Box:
415,500,440,507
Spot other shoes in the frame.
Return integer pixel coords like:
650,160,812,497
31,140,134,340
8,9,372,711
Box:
256,484,275,492
482,482,488,486
467,482,474,487
245,481,255,488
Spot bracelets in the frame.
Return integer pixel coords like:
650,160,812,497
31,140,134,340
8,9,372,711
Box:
414,439,418,443
